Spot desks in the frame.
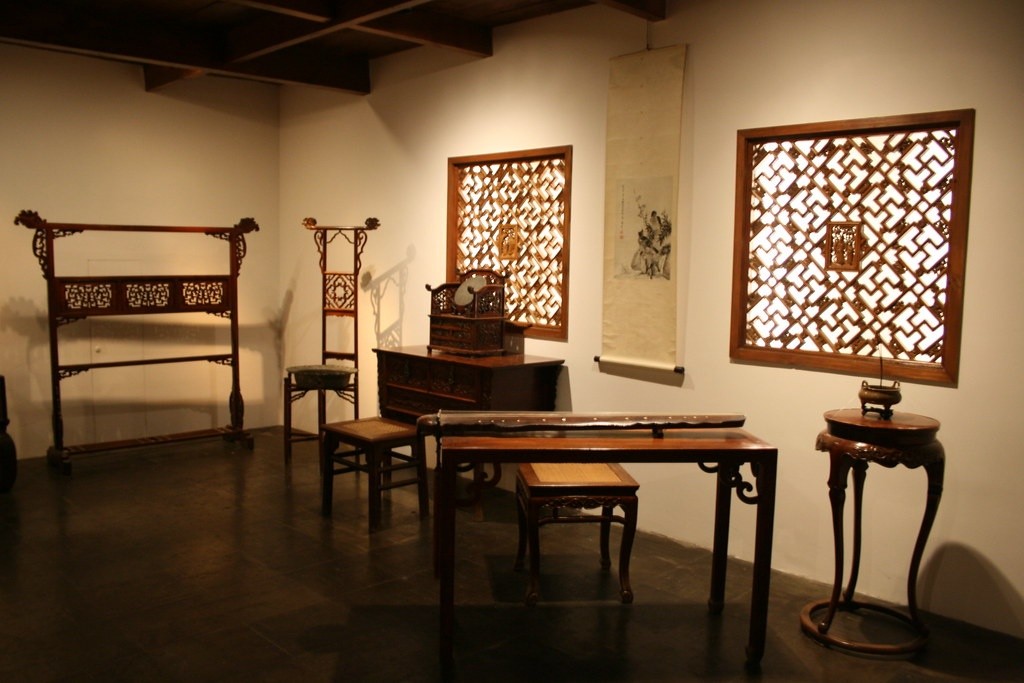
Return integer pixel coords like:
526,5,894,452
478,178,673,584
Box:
434,410,777,665
799,407,946,655
371,346,565,521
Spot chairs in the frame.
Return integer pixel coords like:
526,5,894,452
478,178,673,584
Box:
425,266,512,356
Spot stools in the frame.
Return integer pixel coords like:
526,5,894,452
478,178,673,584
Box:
322,417,428,541
512,463,641,609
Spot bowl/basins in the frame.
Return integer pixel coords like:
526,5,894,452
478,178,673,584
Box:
284,364,357,390
